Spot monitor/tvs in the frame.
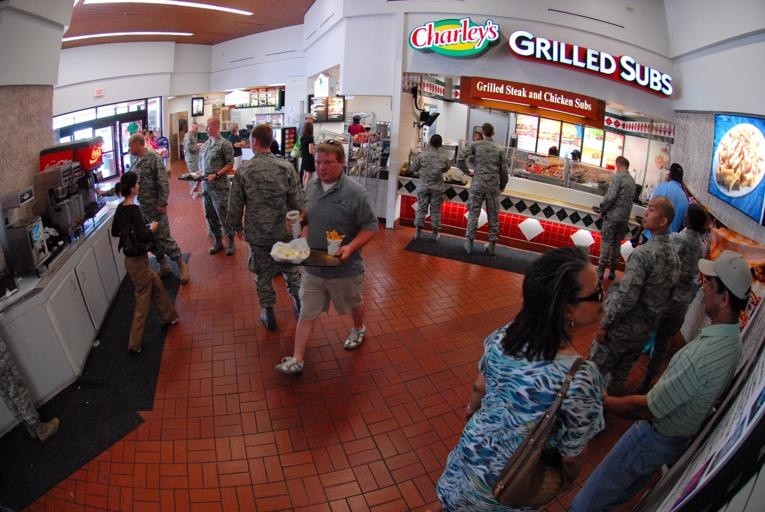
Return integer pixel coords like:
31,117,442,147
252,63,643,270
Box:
307,94,345,122
419,112,440,129
191,98,204,117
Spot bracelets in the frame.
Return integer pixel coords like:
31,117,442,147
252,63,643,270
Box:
467,404,481,412
597,392,612,405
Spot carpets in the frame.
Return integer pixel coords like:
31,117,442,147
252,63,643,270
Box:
513,248,543,274
405,231,513,274
0,370,146,512
82,253,191,411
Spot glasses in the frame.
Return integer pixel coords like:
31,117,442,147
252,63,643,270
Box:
571,281,603,303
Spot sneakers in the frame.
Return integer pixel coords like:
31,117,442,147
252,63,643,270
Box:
159,262,191,283
344,326,365,349
276,357,304,375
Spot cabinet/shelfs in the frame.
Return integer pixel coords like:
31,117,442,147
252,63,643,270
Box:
456,140,615,196
0,214,128,437
345,135,382,208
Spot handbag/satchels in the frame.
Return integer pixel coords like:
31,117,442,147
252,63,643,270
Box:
290,136,302,157
492,358,584,506
123,208,153,257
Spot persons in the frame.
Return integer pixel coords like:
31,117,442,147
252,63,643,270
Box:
97,113,317,201
408,134,452,244
637,203,713,397
270,138,380,376
585,193,683,396
632,162,689,249
1,318,64,445
347,114,368,148
465,130,483,170
456,123,510,256
221,125,305,333
109,170,180,354
563,248,755,512
436,244,610,512
548,145,560,157
127,133,190,285
190,117,236,256
593,155,638,281
571,149,582,162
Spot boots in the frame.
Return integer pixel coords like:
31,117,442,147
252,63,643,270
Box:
415,228,440,240
209,237,234,256
260,308,276,331
464,239,495,256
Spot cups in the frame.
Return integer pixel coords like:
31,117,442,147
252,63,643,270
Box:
286,210,303,239
327,236,343,257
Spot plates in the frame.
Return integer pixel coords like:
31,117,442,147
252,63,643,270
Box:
713,122,760,198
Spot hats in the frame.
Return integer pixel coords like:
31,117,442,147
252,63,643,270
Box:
571,150,580,154
305,113,317,120
698,250,752,299
271,141,282,154
